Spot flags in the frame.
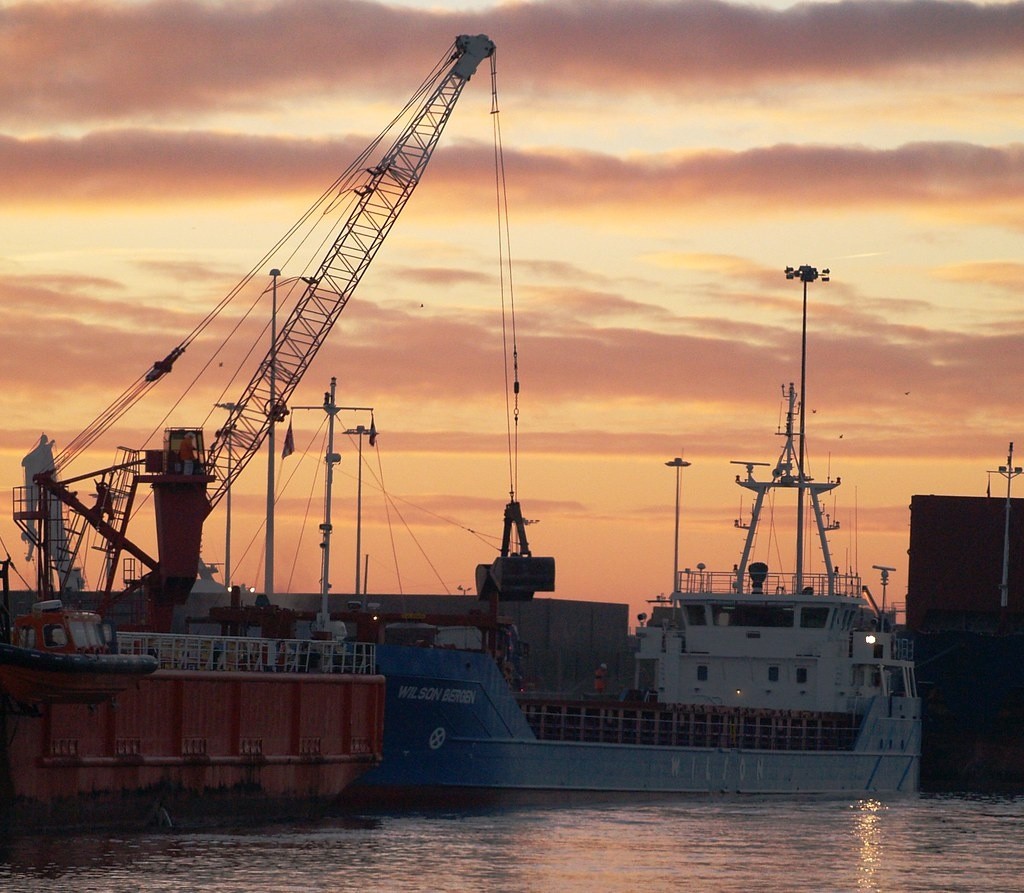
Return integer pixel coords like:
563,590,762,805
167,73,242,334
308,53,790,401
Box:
281,420,295,460
369,414,380,447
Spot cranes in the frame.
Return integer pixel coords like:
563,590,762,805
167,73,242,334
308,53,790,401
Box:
14,28,554,662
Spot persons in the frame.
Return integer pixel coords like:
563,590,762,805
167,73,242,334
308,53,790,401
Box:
181,432,197,476
594,664,608,696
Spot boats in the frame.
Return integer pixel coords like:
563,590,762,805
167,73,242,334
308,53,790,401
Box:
387,623,531,689
391,258,926,804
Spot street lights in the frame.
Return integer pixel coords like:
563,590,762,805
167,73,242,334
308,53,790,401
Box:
985,442,1024,605
